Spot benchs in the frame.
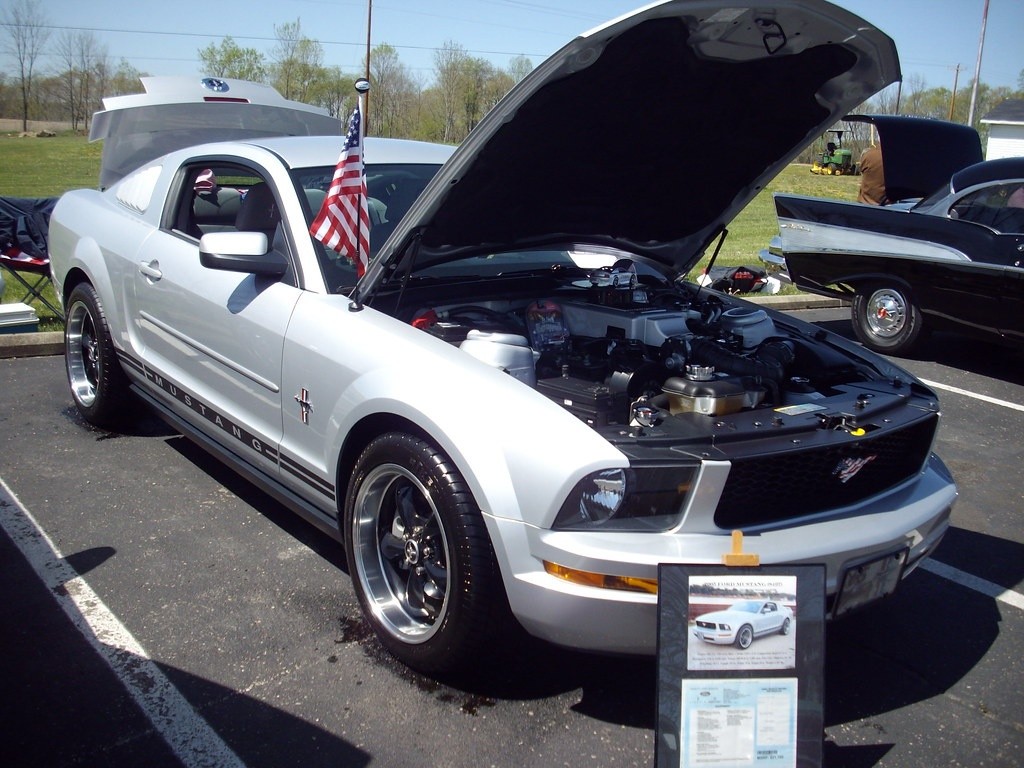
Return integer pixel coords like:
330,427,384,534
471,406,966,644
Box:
192,187,388,235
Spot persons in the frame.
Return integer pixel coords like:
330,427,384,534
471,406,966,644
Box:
856,135,897,206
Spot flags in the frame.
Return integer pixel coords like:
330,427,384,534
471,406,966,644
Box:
310,108,370,281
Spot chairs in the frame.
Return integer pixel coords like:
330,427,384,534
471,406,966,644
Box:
235,181,276,240
370,177,422,259
0,197,65,322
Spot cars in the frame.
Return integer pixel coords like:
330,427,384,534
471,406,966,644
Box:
756,112,1024,356
44,0,958,683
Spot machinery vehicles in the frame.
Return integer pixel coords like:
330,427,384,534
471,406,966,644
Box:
809,128,853,177
